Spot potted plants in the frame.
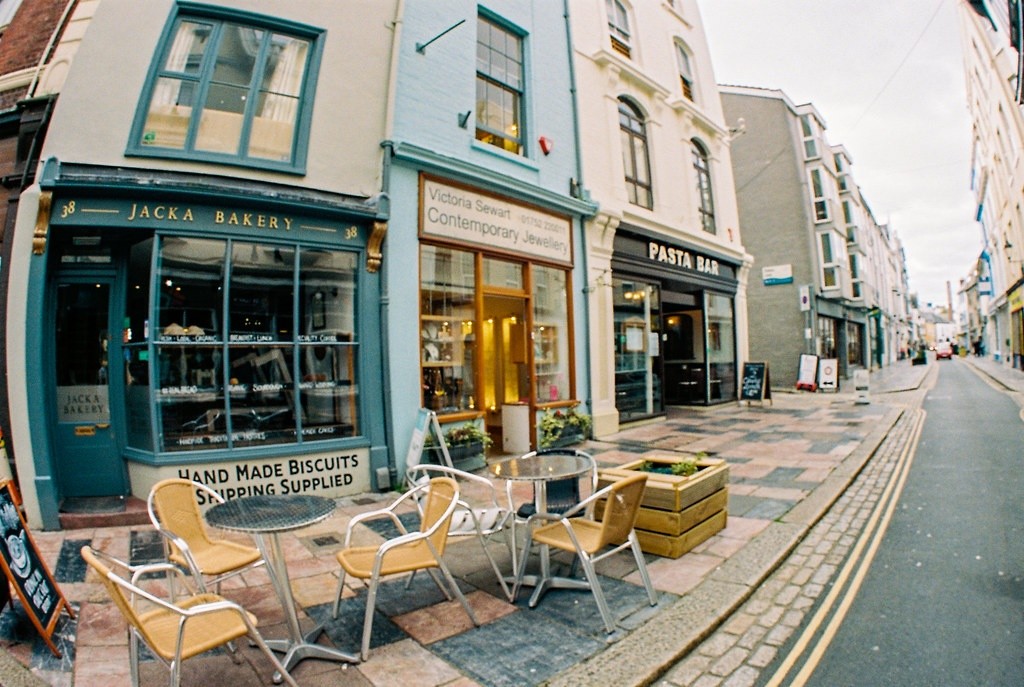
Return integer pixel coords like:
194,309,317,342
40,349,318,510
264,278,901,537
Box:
417,423,494,472
596,453,731,559
530,399,591,449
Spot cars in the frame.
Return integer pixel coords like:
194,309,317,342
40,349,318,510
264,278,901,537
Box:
935,342,953,360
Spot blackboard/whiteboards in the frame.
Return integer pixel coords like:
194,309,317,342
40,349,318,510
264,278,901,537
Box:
0,478,66,642
737,360,771,400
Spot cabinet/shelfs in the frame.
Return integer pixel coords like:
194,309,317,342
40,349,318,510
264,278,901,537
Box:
417,315,476,424
122,340,358,466
510,322,560,411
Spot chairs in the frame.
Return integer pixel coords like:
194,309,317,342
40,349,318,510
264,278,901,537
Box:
80,545,300,687
505,447,599,581
405,463,522,601
332,476,482,662
511,473,658,634
147,478,300,654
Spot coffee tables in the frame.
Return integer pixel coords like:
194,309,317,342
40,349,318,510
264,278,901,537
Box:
205,494,361,684
489,456,595,608
